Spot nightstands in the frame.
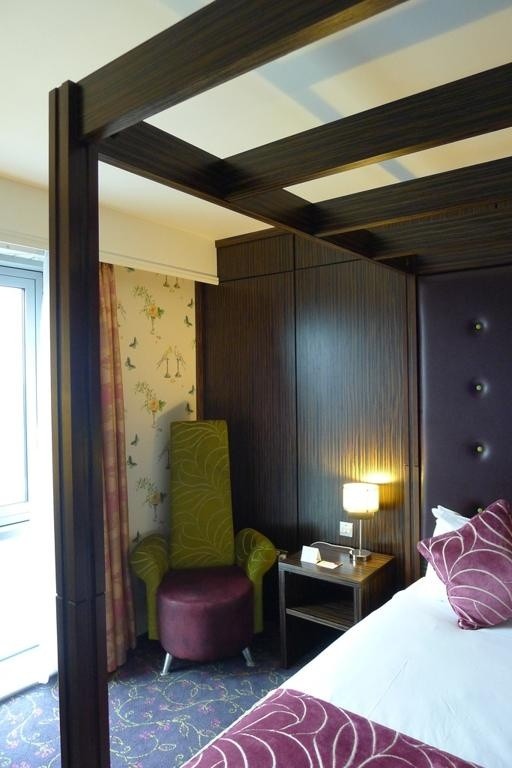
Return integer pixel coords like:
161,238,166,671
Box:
278,542,396,671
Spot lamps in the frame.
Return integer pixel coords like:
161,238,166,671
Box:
342,482,380,562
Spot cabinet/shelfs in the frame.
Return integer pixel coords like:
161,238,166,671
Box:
194,227,420,622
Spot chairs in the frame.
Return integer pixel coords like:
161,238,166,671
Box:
127,419,278,677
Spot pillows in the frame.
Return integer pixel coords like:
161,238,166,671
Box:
417,499,512,629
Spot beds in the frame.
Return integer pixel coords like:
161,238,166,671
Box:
179,575,511,768
